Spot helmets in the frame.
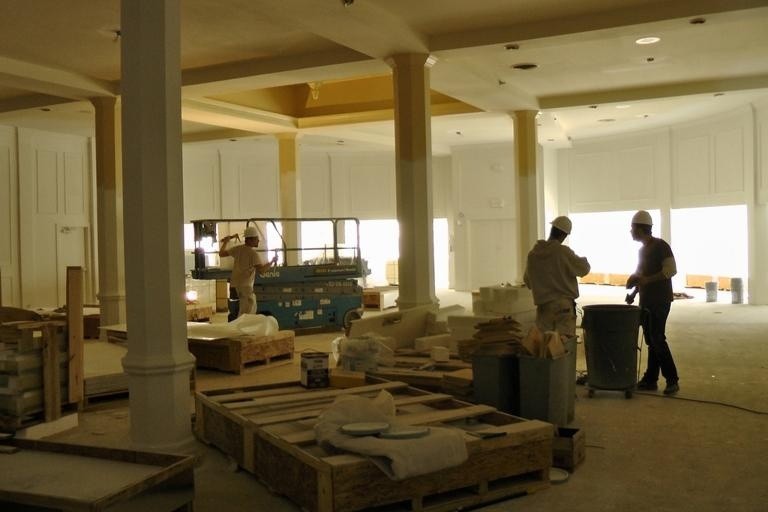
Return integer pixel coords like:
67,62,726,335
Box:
549,216,572,234
242,227,260,237
632,210,652,225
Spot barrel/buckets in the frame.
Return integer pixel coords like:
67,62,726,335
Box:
705,281,717,302
731,278,743,304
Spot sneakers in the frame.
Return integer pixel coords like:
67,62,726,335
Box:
663,377,680,394
637,378,657,390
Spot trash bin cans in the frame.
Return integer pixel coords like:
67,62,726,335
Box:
731,278,742,305
472,333,578,428
581,305,643,400
706,282,717,302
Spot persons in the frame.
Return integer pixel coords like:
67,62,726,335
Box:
626,210,680,396
523,214,590,334
219,227,276,319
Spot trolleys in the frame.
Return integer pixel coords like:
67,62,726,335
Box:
192,217,371,328
